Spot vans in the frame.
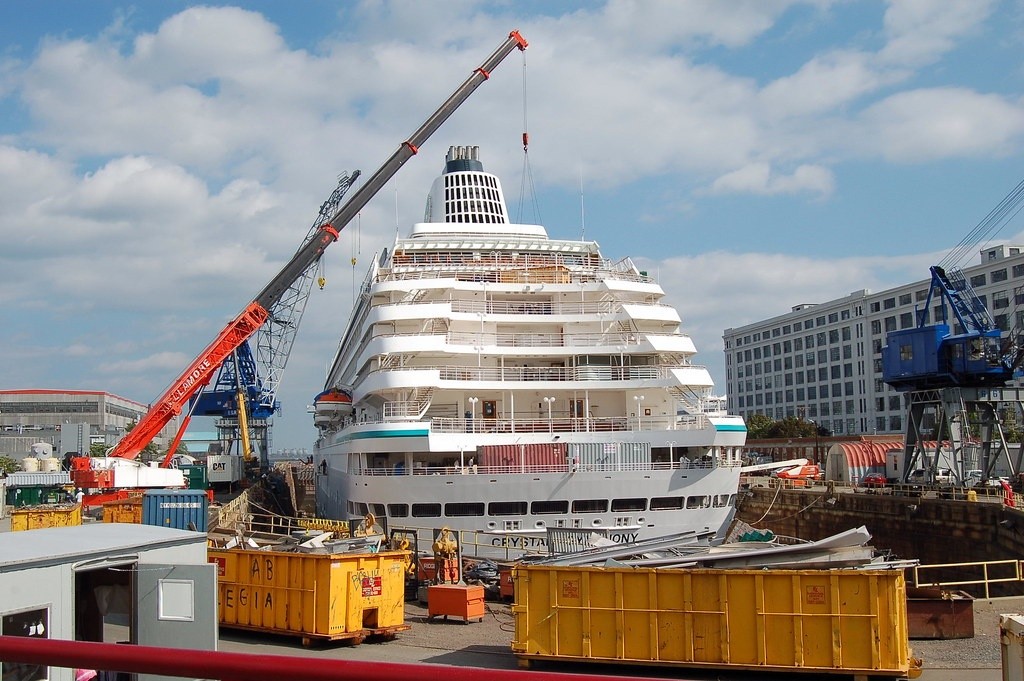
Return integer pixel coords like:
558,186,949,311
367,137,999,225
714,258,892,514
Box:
908,467,958,485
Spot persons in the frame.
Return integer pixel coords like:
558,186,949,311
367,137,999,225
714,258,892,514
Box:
320,459,327,475
656,454,711,468
66,487,85,516
329,411,356,432
464,412,473,433
448,456,475,475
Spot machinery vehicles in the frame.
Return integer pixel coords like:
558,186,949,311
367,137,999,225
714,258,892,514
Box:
69,30,530,518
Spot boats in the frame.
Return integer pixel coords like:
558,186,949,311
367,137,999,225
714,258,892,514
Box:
314,388,353,415
314,412,342,427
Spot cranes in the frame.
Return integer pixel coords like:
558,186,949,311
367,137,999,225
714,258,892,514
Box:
187,168,363,469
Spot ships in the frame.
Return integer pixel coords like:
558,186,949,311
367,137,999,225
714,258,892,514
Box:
307,145,747,560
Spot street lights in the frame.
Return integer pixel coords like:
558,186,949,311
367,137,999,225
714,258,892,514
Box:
543,396,556,434
634,396,645,431
469,397,478,433
806,418,819,463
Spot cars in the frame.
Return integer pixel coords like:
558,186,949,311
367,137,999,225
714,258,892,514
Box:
863,473,887,488
964,469,982,480
771,466,790,479
